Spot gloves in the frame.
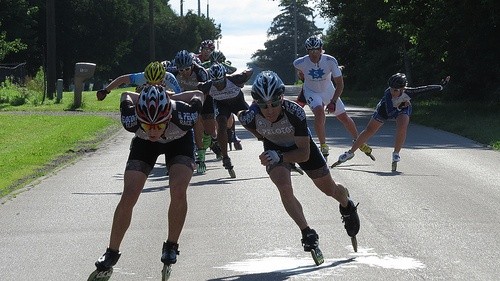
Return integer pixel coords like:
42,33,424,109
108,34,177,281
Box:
96,88,110,100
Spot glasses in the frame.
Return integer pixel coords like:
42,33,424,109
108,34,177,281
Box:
257,100,281,109
138,119,169,133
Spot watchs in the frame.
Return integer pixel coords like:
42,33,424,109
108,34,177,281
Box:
277,150,284,163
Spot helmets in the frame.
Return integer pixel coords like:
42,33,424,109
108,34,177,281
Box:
388,73,407,88
305,35,323,50
208,50,226,64
174,50,193,68
134,84,172,125
161,60,178,72
144,61,166,84
201,40,215,51
209,64,226,81
251,71,286,104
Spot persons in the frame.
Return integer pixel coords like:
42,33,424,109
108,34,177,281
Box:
97,40,253,179
331,73,450,172
238,71,361,265
87,84,204,281
296,65,345,106
293,35,375,161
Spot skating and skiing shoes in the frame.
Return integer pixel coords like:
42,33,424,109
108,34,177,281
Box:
391,152,400,171
321,143,330,162
301,229,324,266
161,242,180,281
195,149,206,175
203,132,212,148
339,199,360,252
331,150,354,169
95,248,121,278
223,156,237,178
233,136,243,151
353,138,376,162
